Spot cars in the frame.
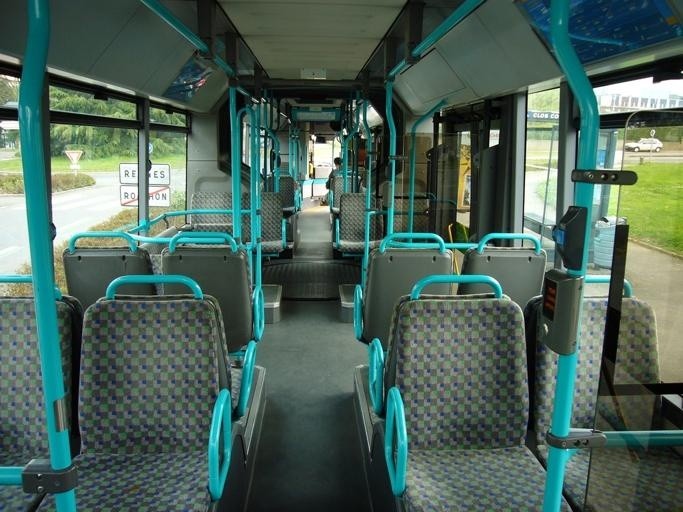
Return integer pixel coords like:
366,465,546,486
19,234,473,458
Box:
625,138,663,152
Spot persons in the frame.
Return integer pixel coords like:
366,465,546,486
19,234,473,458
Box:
318,157,342,204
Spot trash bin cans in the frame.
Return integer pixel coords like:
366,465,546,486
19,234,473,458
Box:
598,149,606,166
593,215,627,270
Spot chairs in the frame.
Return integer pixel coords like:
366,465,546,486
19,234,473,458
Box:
0,273,258,512
64,230,265,358
353,274,682,511
352,231,548,351
125,190,289,255
327,167,436,256
266,161,304,216
